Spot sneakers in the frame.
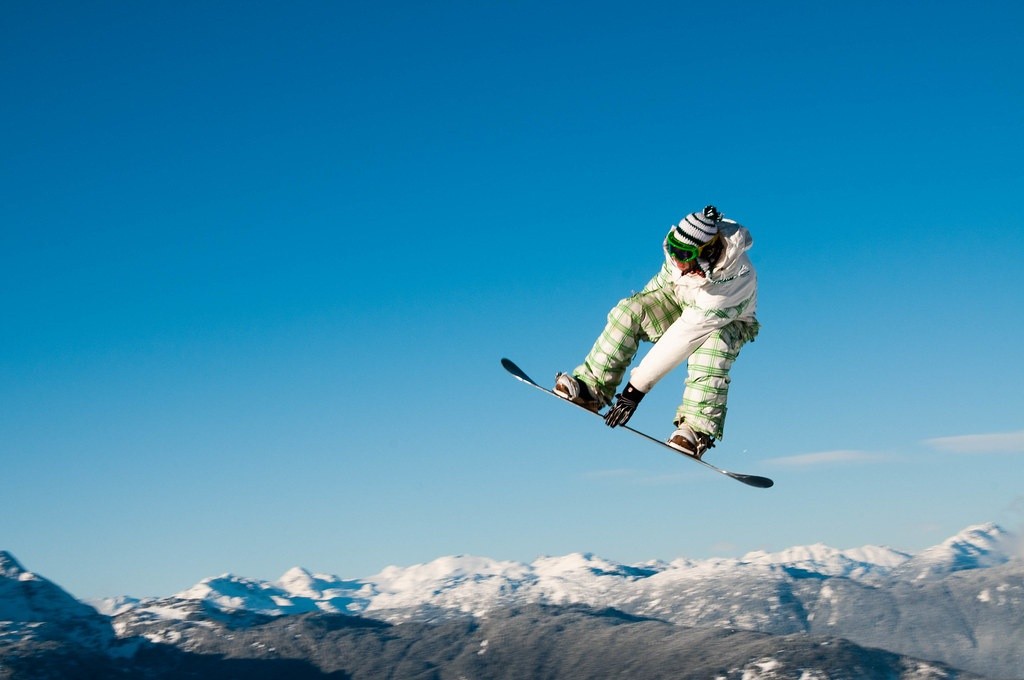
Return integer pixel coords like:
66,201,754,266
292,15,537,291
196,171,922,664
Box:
667,424,714,458
552,372,609,414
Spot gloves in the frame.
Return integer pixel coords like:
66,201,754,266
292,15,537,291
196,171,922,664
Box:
602,394,636,427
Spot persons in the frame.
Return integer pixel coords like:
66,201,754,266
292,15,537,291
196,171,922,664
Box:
552,204,762,458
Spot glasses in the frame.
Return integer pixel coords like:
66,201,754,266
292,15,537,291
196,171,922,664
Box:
666,232,699,260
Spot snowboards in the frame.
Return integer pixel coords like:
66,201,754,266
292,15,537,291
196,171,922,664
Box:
500,356,776,492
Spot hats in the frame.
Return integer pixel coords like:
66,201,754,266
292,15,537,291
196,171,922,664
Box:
670,204,722,251
